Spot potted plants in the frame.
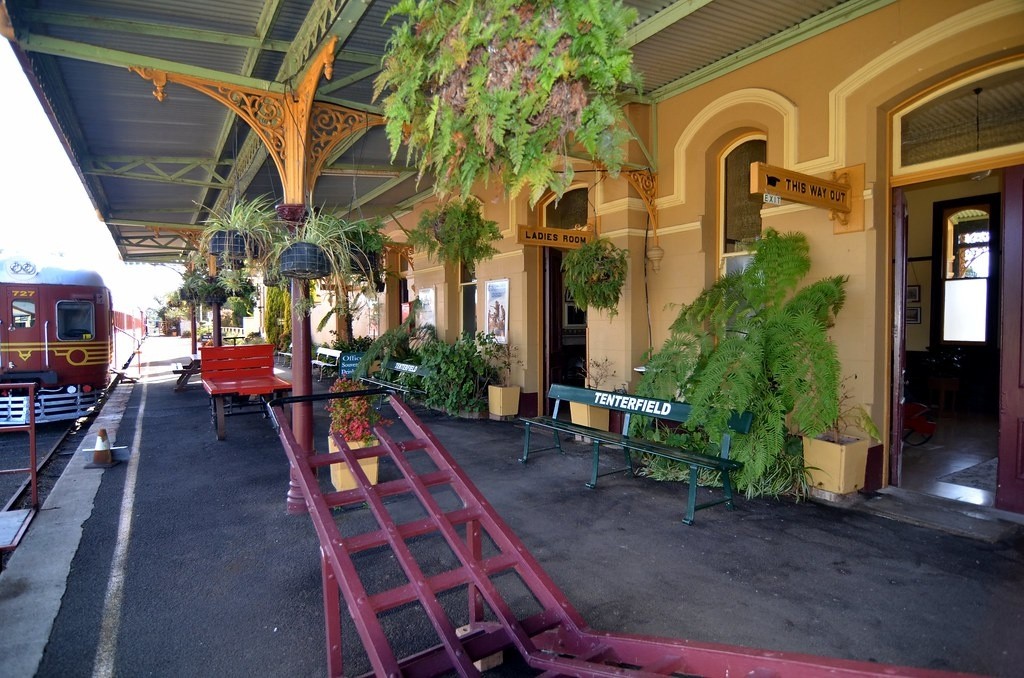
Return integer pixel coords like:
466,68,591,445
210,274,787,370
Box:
800,371,884,493
176,190,396,304
558,234,631,323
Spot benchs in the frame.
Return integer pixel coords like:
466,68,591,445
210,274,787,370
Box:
360,359,431,421
312,347,343,382
518,383,755,525
276,342,293,369
199,344,292,437
171,362,184,373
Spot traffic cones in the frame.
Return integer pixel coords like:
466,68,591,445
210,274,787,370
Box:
83,426,122,468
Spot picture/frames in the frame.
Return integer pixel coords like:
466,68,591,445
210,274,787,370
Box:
906,285,920,302
906,306,922,324
484,278,511,345
418,287,437,337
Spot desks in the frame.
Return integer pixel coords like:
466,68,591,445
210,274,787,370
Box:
172,353,202,391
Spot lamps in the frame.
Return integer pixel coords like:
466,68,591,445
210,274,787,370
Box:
969,87,992,180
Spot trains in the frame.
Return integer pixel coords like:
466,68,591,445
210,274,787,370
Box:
0,259,148,426
